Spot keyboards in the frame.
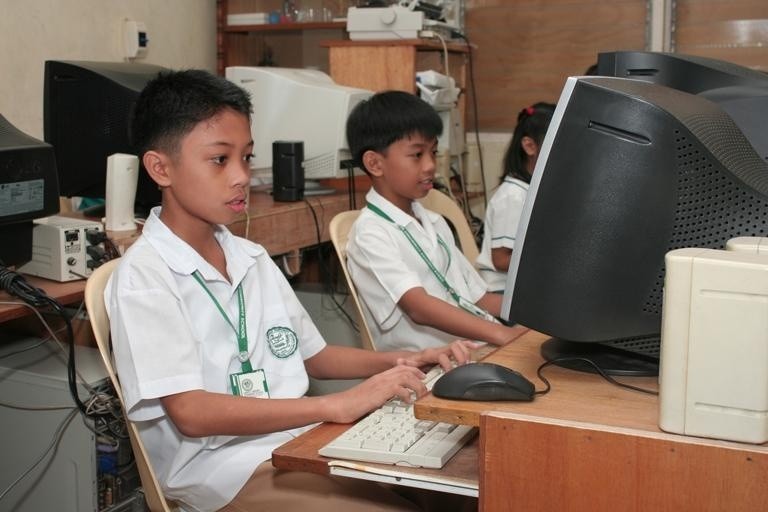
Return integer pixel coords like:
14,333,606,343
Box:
318,359,479,469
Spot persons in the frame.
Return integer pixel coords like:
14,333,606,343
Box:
345,89,528,360
105,66,481,511
476,101,562,328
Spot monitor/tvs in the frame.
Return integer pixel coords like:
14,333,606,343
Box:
0,113,60,266
43,58,181,220
225,66,375,188
500,76,768,377
597,51,768,161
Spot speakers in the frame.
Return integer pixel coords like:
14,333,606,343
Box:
104,153,139,231
272,140,306,202
726,236,768,253
660,247,768,445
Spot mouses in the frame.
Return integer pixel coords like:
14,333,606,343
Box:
432,364,535,401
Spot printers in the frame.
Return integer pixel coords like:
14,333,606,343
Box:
346,0,459,42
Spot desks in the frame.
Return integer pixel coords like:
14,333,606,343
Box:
271,322,767,512
0,167,366,327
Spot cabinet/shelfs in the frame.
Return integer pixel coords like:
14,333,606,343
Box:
213,0,350,78
317,39,479,154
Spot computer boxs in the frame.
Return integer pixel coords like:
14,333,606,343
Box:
7,215,106,283
0,337,147,512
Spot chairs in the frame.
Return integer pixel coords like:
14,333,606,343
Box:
413,186,481,269
81,256,180,511
327,208,377,356
